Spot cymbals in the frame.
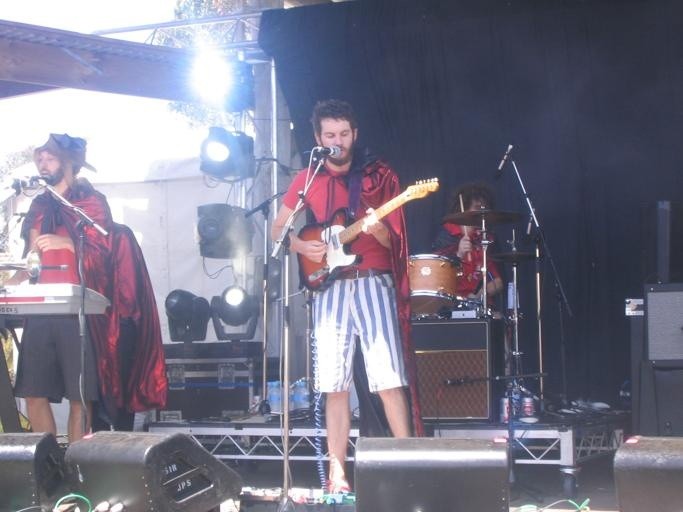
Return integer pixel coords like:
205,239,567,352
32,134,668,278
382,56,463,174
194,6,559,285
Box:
491,250,537,263
444,210,524,228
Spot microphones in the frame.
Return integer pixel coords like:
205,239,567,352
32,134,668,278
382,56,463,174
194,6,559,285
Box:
444,379,457,385
39,175,50,189
315,146,340,159
495,144,514,176
525,208,536,236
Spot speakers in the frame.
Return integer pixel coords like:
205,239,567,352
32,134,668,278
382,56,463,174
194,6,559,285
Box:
0,432,65,512
612,435,683,512
64,431,242,512
354,438,510,512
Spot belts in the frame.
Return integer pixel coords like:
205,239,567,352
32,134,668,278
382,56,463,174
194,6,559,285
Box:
337,267,390,278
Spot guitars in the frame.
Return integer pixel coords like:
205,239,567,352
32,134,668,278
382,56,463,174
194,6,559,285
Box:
296,177,440,290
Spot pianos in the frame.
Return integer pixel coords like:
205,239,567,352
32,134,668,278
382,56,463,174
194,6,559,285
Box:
0,282,111,316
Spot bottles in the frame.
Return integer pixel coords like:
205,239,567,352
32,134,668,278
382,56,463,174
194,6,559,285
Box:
265,375,309,414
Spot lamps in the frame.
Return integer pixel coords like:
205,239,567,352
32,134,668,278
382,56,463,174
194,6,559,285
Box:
164,61,259,344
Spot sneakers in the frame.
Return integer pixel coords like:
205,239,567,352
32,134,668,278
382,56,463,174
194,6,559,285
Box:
328,481,351,494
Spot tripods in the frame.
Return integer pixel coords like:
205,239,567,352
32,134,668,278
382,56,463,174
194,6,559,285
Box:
512,157,617,416
221,188,315,422
456,372,549,503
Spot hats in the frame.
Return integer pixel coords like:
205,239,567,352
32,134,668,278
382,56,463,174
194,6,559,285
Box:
32,134,95,171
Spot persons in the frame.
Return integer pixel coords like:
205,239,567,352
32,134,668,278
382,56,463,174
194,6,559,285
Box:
1,133,118,449
429,181,505,311
270,98,426,495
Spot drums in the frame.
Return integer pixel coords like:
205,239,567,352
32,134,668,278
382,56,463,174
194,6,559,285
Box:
407,253,460,316
